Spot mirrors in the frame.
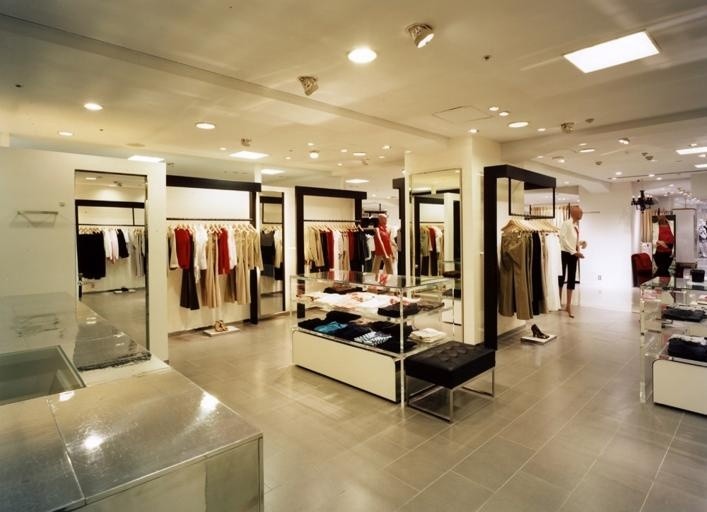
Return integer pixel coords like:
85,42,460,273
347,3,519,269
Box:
409,167,464,343
73,170,149,353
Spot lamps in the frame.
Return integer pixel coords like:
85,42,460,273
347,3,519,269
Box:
631,189,657,214
240,138,253,147
406,22,436,48
297,75,319,96
617,136,630,145
560,122,575,134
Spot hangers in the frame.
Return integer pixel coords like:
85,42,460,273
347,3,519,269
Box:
500,203,579,234
419,220,443,229
78,224,146,235
304,219,359,234
166,217,283,237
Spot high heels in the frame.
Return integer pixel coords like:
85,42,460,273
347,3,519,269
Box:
531,324,548,339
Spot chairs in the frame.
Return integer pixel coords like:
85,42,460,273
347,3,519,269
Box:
632,253,654,313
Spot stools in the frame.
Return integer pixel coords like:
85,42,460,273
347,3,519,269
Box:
404,341,496,424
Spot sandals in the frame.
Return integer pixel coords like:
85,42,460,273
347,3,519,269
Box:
219,320,228,331
215,321,223,332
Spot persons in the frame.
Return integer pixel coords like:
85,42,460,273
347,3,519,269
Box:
373,213,400,276
557,204,587,318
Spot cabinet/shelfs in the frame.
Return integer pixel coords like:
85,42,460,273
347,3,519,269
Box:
651,334,707,416
640,275,707,403
290,268,454,330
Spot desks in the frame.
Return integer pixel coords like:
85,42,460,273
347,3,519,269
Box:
291,316,452,403
675,261,697,278
73,301,149,370
